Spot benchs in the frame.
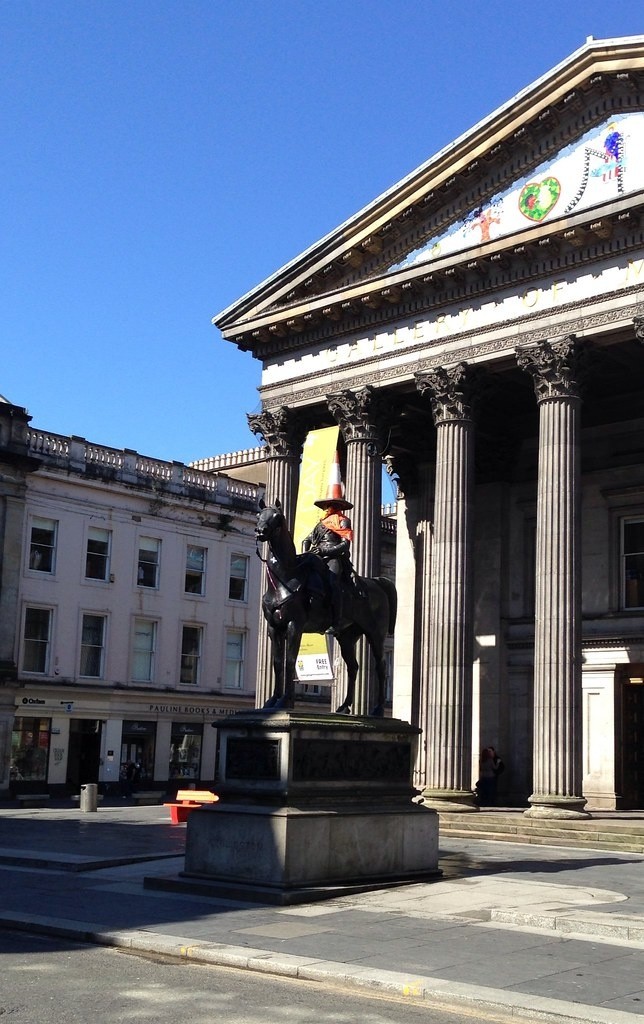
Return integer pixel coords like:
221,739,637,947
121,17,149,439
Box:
164,790,219,824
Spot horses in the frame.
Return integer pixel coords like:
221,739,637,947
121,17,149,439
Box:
254,497,398,718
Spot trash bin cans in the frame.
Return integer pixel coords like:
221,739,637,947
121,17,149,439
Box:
79,783,98,813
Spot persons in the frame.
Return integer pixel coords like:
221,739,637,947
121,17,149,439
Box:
476,747,507,806
299,450,357,638
